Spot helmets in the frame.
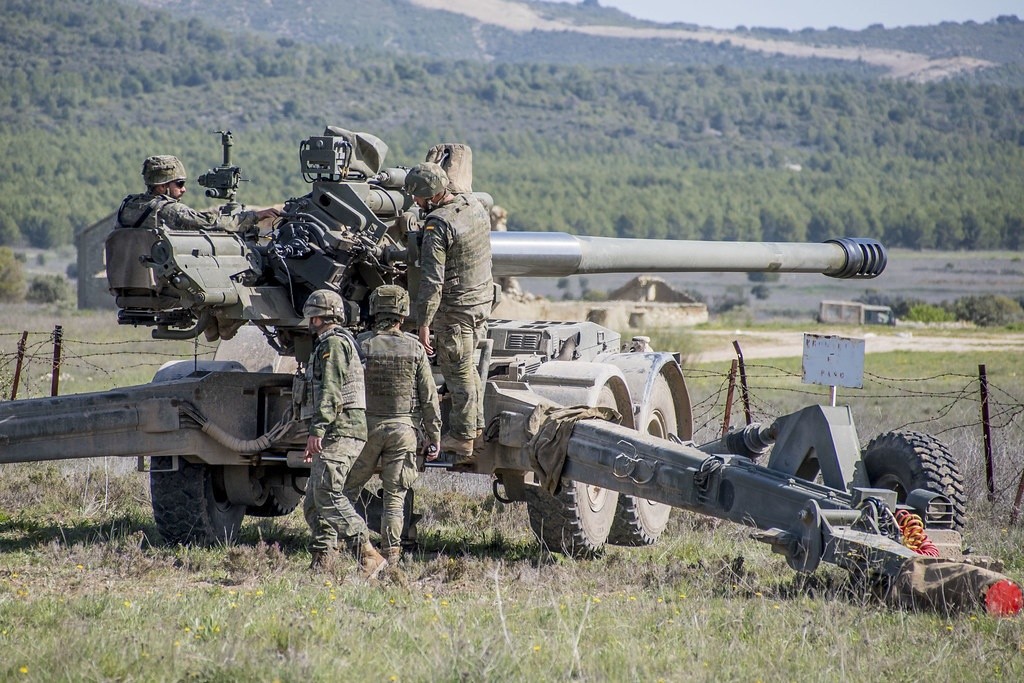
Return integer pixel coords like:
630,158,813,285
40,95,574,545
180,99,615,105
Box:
403,162,450,198
141,155,187,186
303,289,345,322
368,283,411,318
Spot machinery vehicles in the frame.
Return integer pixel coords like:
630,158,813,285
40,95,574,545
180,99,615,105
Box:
7,124,1023,624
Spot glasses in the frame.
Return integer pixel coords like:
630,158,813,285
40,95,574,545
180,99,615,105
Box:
173,180,186,189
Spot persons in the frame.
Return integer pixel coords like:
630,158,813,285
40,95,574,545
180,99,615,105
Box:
301,288,389,586
406,161,492,457
115,156,280,342
338,285,442,574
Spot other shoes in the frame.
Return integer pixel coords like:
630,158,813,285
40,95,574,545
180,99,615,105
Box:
306,550,338,577
341,541,388,590
440,430,474,457
380,545,402,574
472,428,486,450
